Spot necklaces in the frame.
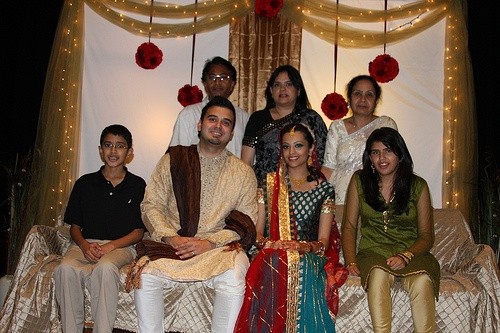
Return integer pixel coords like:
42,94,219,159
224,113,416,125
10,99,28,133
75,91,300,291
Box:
288,170,310,186
379,185,396,232
350,116,372,131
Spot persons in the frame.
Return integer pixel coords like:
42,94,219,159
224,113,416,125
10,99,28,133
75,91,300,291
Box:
340,128,440,333
320,75,399,205
232,122,349,333
55,124,147,333
168,55,256,168
125,94,257,333
240,65,327,182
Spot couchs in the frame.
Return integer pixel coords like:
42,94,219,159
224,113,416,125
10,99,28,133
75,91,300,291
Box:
0,208,500,333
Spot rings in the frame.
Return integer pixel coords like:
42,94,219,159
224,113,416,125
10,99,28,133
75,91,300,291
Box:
192,251,195,256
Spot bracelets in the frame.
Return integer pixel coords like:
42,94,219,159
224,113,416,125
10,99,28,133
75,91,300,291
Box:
396,249,415,266
255,237,282,250
298,241,326,256
346,262,359,269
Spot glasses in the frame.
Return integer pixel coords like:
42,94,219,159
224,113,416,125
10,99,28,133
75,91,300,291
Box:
207,75,231,82
